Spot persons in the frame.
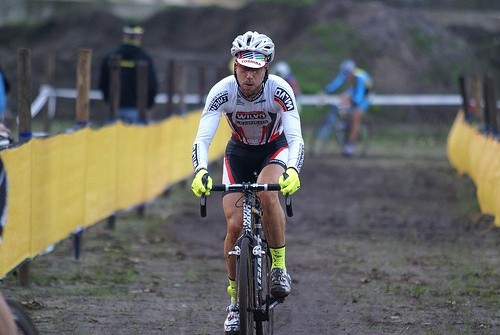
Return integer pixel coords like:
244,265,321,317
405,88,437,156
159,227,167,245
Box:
0,63,39,335
274,61,301,115
191,31,305,332
98,19,157,126
325,60,374,156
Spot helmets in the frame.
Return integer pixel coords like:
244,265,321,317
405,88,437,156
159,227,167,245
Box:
341,60,355,74
230,31,275,64
274,62,290,78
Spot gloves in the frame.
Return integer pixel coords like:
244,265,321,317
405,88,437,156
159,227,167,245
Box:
191,169,212,198
279,168,300,196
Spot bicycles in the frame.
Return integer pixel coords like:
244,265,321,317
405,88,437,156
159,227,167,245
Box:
198,173,293,333
308,98,375,162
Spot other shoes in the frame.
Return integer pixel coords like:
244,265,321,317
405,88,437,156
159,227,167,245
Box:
343,144,354,155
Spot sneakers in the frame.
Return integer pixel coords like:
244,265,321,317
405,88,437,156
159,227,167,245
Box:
270,268,292,297
223,302,241,335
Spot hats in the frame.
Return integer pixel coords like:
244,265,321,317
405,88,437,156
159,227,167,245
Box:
237,50,267,68
